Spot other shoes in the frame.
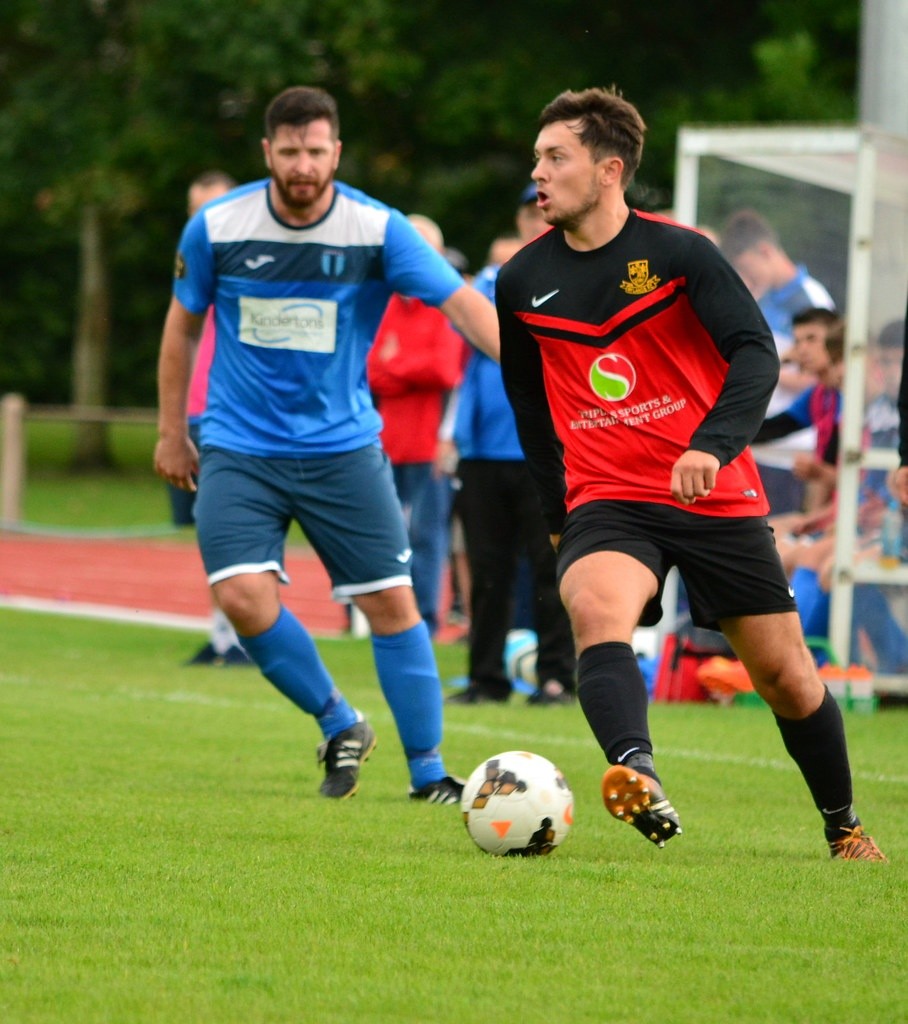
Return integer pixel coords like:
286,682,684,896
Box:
192,640,218,664
526,680,572,705
209,647,249,665
443,690,488,705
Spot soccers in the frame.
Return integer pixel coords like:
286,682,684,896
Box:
461,749,575,857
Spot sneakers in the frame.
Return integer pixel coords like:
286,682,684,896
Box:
602,765,682,849
409,773,465,805
823,816,888,863
315,708,379,799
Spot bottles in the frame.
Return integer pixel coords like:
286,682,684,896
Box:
880,500,903,568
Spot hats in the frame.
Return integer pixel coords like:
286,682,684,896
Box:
519,183,540,204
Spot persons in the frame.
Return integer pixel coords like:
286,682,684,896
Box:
365,210,474,638
167,157,255,672
497,84,889,863
346,187,908,672
152,92,499,803
435,172,577,704
